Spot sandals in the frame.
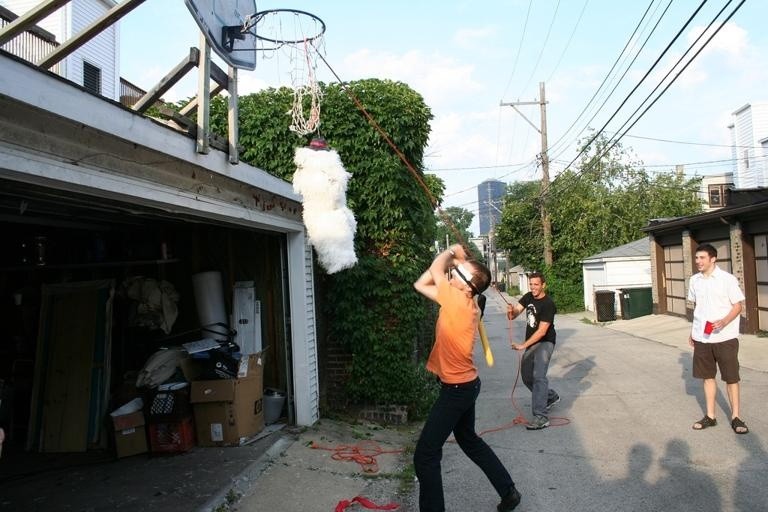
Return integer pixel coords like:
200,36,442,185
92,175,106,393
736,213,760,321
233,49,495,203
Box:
692,415,717,430
732,417,749,434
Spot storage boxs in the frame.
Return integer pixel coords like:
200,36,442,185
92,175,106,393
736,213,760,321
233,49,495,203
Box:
111,408,148,458
178,344,271,446
147,396,195,455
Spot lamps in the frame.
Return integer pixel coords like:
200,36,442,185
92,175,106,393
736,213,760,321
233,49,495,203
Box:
17,231,49,271
12,288,24,306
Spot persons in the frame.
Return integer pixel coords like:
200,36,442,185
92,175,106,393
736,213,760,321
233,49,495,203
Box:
410,244,522,511
506,271,560,430
685,245,750,434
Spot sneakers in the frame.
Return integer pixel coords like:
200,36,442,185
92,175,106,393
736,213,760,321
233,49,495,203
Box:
526,415,549,430
546,394,560,409
497,487,521,512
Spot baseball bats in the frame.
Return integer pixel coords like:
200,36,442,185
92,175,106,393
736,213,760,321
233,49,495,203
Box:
455,258,494,366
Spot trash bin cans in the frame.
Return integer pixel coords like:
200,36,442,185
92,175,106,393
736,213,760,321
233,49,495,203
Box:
499,283,504,292
595,290,614,322
264,389,285,424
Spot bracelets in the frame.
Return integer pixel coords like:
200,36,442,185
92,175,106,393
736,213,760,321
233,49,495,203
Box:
449,249,455,257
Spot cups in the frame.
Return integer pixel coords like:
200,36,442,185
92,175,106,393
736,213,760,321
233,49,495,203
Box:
704,320,714,335
